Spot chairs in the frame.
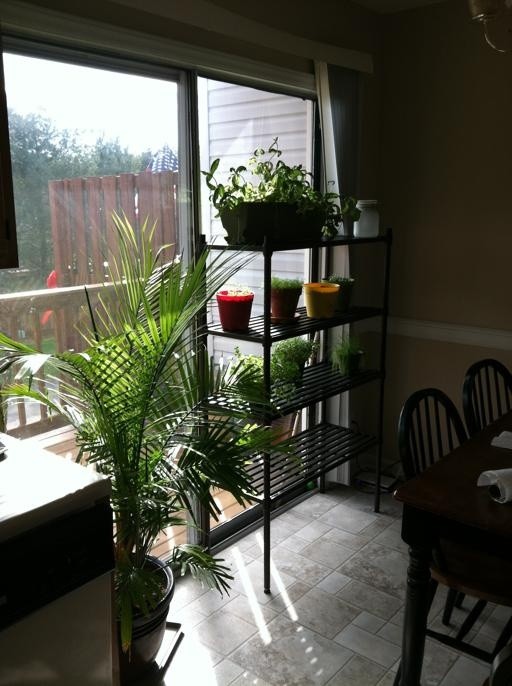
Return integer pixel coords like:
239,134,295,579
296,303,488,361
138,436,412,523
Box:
439,357,512,629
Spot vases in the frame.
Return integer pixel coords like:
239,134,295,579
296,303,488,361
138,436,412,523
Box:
302,282,342,319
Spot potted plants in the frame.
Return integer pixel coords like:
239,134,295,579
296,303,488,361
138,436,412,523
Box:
1,209,295,683
200,132,362,246
271,275,304,318
321,275,355,308
214,286,255,332
229,335,364,406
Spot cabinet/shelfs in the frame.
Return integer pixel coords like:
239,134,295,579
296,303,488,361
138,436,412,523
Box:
1,480,119,686
196,219,392,593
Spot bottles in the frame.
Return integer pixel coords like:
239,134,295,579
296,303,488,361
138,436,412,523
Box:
353,199,381,237
